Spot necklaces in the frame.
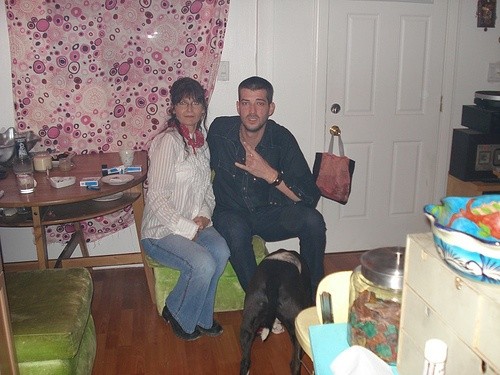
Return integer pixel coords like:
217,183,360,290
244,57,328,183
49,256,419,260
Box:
172,120,205,151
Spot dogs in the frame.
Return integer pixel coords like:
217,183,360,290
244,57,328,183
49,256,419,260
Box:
239,249,311,375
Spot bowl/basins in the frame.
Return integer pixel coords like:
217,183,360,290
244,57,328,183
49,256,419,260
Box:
422,210,500,285
0,145,15,163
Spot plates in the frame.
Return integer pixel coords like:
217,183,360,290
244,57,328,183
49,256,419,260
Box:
101,173,134,186
92,192,122,202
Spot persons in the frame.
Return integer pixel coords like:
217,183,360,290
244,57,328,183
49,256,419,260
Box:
204,77,327,333
139,76,230,341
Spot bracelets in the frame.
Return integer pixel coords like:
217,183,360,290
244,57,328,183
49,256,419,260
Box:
271,172,283,187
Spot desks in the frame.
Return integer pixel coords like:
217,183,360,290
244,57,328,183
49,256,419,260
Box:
294,308,323,375
0,148,158,306
446,175,500,198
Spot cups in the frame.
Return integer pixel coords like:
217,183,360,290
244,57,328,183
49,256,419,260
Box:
119,149,135,165
13,169,35,195
32,154,52,173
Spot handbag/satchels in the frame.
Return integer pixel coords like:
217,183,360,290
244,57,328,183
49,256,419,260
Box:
312,130,356,206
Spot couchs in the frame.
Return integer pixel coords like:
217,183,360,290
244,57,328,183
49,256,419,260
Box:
0,266,98,375
145,171,267,317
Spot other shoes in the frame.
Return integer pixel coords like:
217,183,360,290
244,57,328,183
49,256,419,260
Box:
197,319,223,339
161,304,201,341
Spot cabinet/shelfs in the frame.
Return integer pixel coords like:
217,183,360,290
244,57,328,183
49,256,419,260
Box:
395,232,500,375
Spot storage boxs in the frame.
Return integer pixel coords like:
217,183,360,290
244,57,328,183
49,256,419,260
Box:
104,165,125,174
80,175,101,188
127,165,142,172
